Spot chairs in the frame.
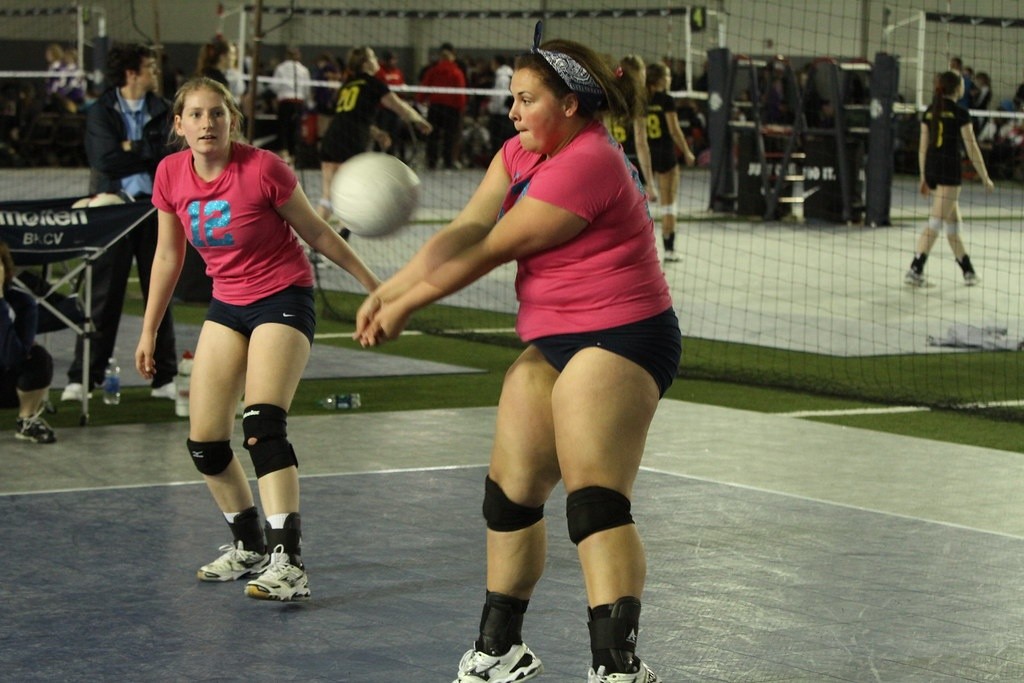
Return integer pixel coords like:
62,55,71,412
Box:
14,253,93,425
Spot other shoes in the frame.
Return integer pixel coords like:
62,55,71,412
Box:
151,381,176,398
964,270,979,284
61,381,93,400
904,272,936,288
664,250,684,260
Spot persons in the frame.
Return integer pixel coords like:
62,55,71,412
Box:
602,56,657,202
645,61,695,262
311,46,433,270
243,43,514,169
0,243,55,443
268,47,310,169
41,45,84,109
950,59,1024,181
350,39,682,683
198,35,236,91
134,79,379,601
62,42,177,400
905,70,994,287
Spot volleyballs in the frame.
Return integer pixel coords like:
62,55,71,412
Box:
330,151,424,238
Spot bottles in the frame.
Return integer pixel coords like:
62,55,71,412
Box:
102,357,121,405
176,349,195,418
313,392,361,411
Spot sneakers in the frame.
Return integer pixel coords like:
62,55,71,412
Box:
453,639,544,683
586,656,661,683
244,543,311,600
198,539,272,580
15,406,56,442
